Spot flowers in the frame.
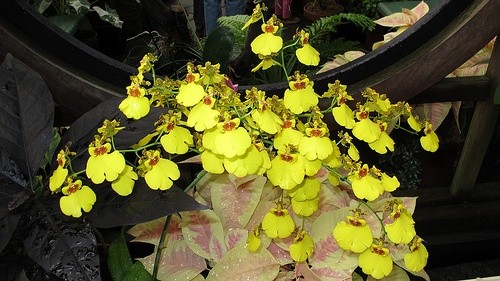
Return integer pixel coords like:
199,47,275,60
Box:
165,2,429,87
35,2,438,281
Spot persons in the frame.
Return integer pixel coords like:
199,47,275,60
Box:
193,0,248,39
203,26,235,67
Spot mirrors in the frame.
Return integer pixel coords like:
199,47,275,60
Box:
9,0,456,90
